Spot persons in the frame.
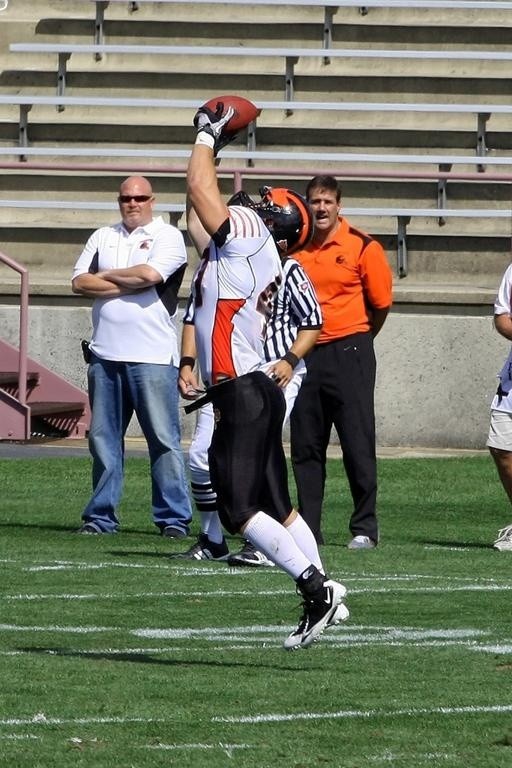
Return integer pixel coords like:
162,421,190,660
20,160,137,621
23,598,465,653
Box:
484,262,512,553
277,173,395,552
172,100,353,654
173,251,323,569
70,175,196,540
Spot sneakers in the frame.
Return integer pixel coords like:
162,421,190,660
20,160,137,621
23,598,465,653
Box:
226,538,277,568
79,525,100,536
282,600,350,651
492,523,512,552
169,531,231,561
164,527,186,538
345,533,380,551
295,563,348,647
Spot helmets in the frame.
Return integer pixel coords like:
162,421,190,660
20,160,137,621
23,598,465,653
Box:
226,184,316,259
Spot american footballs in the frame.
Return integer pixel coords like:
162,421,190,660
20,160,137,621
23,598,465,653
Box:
193,96,260,131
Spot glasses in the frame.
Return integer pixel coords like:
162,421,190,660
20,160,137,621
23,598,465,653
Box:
118,196,151,203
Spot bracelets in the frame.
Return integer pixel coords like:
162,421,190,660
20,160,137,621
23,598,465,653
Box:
178,357,195,370
194,130,217,150
281,352,300,370
